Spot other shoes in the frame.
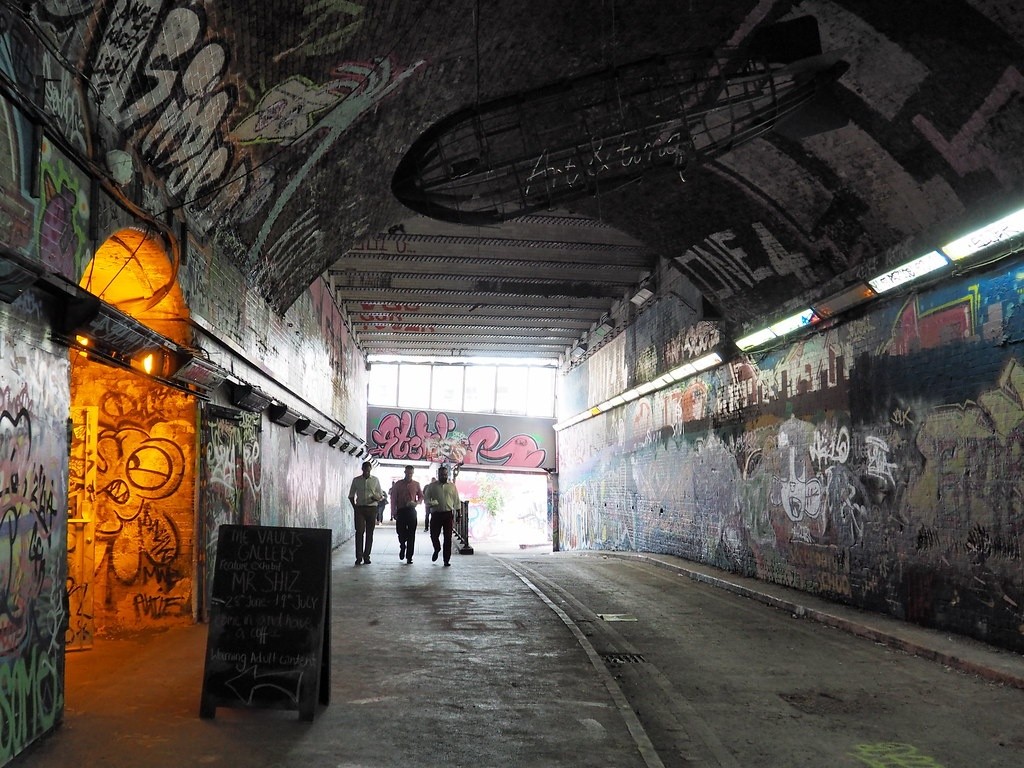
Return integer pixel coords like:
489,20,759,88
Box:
400,548,405,560
390,518,392,521
407,558,413,565
432,547,441,562
444,562,452,567
424,528,427,532
355,558,371,565
375,519,382,526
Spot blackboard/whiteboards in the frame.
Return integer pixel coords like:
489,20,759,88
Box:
198,522,335,713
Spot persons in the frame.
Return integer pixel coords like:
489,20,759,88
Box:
424,466,461,566
423,477,437,532
392,465,424,564
348,461,382,564
376,490,389,525
388,481,396,521
